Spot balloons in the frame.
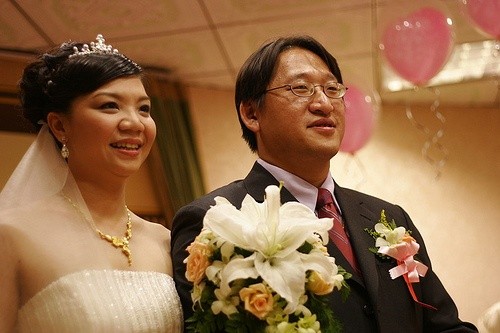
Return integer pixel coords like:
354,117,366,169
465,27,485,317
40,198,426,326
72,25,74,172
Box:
380,6,453,89
463,0,500,42
337,82,374,157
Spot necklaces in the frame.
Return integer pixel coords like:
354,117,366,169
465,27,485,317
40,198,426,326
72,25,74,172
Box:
55,190,133,269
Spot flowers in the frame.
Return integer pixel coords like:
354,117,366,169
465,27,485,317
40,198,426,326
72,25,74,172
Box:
183,181,352,332
363,209,441,312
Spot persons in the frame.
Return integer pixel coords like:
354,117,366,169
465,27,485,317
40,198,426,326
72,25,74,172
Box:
170,34,480,333
0,34,185,333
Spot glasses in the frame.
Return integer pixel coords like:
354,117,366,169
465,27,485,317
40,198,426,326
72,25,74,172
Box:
248,80,348,99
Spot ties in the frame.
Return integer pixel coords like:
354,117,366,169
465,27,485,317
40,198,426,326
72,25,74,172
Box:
316,188,365,283
